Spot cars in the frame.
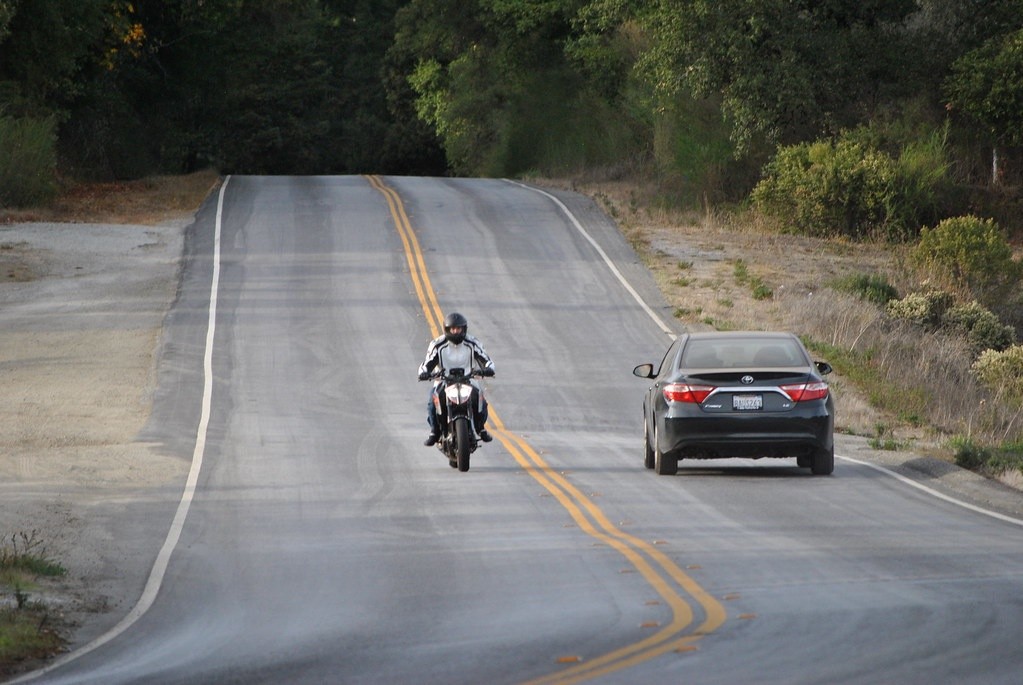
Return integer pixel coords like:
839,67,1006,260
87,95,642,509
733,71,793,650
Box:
633,331,834,475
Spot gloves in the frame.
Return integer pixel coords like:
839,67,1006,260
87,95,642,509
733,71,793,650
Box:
419,373,431,381
482,367,495,377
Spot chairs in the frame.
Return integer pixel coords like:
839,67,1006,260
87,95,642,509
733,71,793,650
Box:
755,347,792,363
689,347,722,367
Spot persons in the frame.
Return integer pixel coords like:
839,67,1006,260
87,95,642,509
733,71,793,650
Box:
419,312,496,446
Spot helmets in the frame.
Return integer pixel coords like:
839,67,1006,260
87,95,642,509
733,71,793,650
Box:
443,313,467,344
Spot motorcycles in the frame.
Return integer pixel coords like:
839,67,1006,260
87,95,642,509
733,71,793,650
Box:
417,368,495,472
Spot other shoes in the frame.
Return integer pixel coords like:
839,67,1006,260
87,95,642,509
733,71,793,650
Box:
478,429,493,442
424,432,441,446
